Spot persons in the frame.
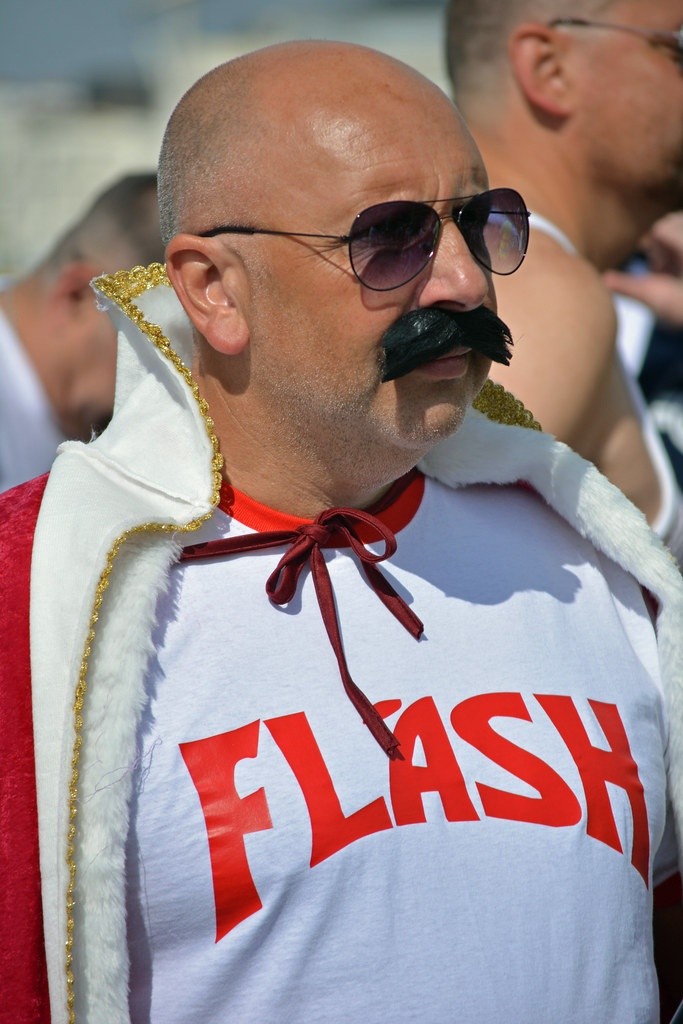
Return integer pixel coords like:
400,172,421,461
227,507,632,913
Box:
1,172,168,495
445,0,683,572
0,40,683,1024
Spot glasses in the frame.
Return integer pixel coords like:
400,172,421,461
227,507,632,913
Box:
198,187,530,292
